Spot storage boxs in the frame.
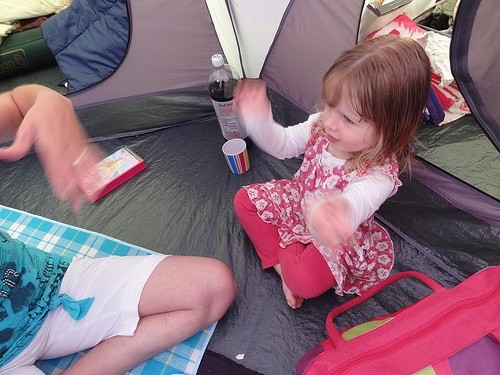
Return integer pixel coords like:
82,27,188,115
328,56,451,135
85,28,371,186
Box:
78,146,147,202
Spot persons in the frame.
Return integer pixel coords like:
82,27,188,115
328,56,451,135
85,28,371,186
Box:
1,83,236,375
234,35,431,310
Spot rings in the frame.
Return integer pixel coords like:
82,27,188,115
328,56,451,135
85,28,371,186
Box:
73,148,87,166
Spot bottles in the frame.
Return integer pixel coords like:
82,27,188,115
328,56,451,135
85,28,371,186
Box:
207,54,251,142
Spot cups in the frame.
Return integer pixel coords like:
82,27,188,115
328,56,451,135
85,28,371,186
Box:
221,138,251,174
